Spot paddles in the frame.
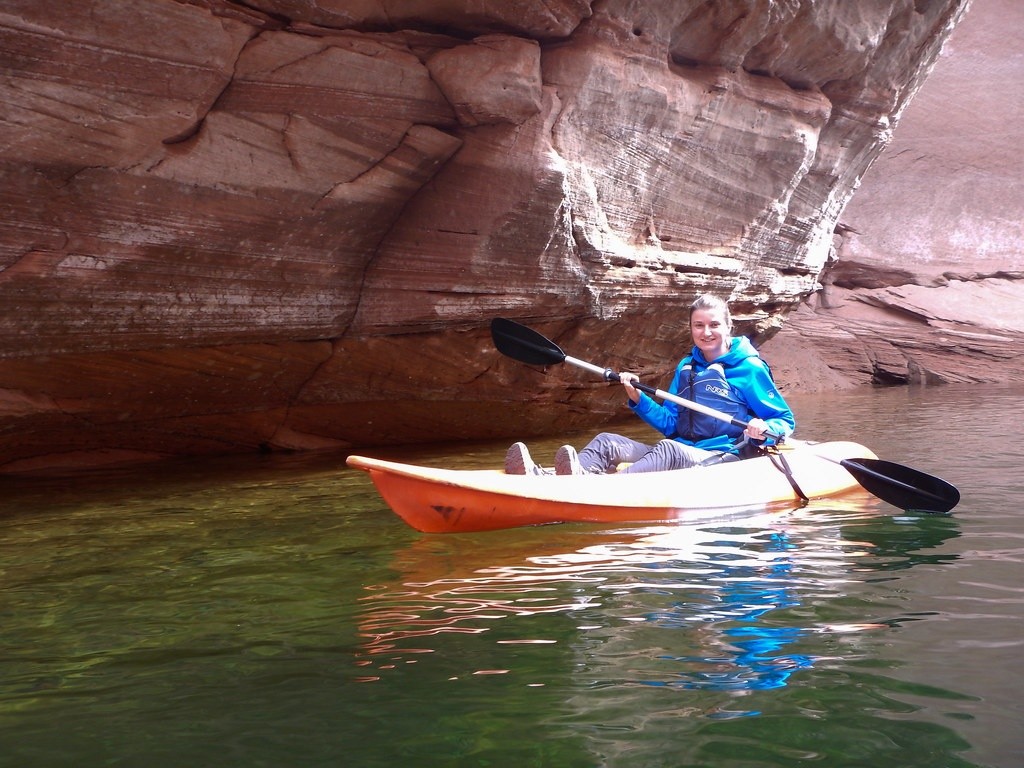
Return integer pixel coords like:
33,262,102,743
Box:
491,314,963,514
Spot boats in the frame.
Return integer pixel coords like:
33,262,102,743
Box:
346,436,879,535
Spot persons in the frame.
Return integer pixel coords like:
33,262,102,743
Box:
505,294,796,476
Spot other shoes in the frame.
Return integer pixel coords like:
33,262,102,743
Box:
555,444,582,476
505,440,550,476
